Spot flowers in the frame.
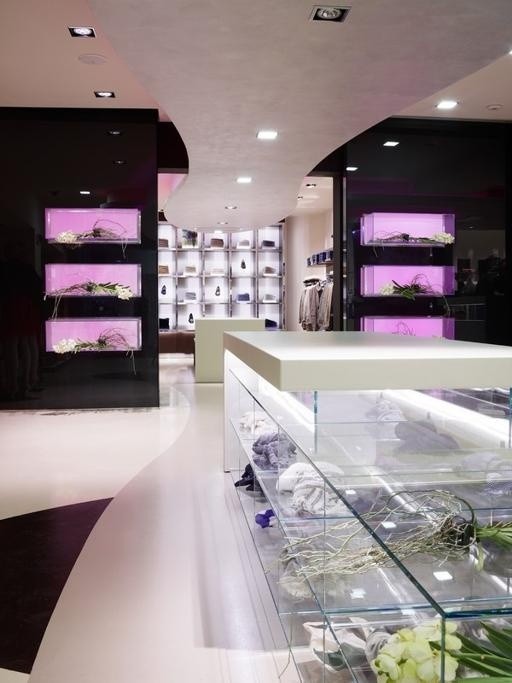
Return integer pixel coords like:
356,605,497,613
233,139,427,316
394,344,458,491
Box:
52,327,138,376
43,277,134,320
371,615,511,683
54,218,129,254
378,272,451,331
372,229,456,258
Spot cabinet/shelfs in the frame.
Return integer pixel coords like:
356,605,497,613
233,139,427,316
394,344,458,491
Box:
157,221,283,333
194,318,266,383
222,330,512,683
